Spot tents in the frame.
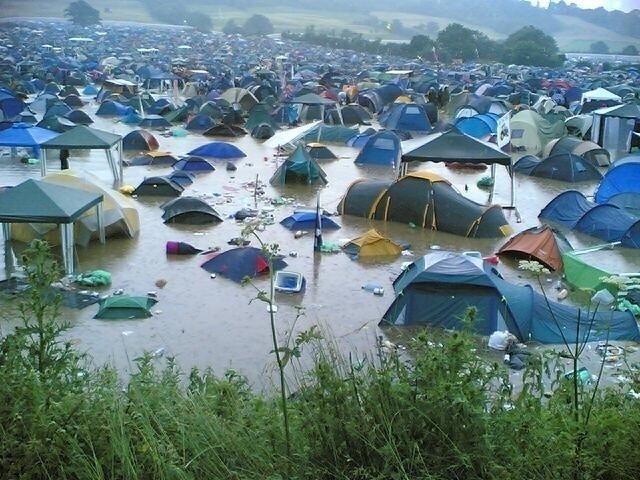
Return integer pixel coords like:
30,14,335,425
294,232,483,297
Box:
93,294,157,320
386,102,434,133
378,252,639,345
454,112,503,137
186,114,217,129
64,110,94,124
163,195,221,223
269,144,326,185
205,125,247,137
251,124,275,139
167,170,194,184
131,175,182,196
337,170,514,240
9,169,140,246
243,111,280,131
97,100,138,115
566,115,595,142
163,128,191,137
1,123,59,177
2,88,25,129
327,104,372,124
344,230,400,255
497,225,576,275
591,103,640,153
221,111,246,125
0,18,640,117
139,115,172,127
540,192,638,250
121,114,142,125
199,246,286,281
303,143,335,159
280,211,340,230
513,154,604,181
296,123,359,143
121,130,159,150
131,152,180,164
40,125,123,184
64,95,87,107
544,137,611,167
395,126,514,208
594,156,640,213
284,93,346,128
346,134,373,146
188,142,247,158
502,111,565,154
1,179,104,275
36,116,78,131
171,156,215,171
165,109,190,123
16,93,73,130
11,105,44,124
